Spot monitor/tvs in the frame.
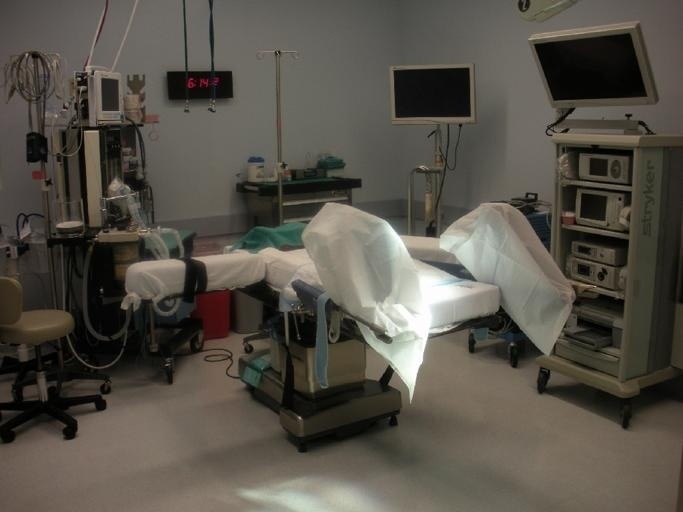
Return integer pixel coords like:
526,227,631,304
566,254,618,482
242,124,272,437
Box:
388,63,477,125
527,20,659,108
94,71,124,121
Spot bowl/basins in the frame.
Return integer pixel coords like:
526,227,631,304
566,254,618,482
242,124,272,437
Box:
55,220,83,239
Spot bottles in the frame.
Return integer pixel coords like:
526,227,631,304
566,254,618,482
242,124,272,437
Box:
122,145,136,177
246,156,265,184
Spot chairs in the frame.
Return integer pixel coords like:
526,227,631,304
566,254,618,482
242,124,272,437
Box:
0,275,107,442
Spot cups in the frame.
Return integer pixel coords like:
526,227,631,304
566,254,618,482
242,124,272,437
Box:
123,111,143,124
123,94,140,110
50,197,85,239
561,211,575,225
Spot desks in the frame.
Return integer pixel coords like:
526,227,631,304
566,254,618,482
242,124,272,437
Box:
234,176,363,231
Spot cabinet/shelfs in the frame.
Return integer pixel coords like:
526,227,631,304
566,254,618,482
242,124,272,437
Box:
536,132,683,424
